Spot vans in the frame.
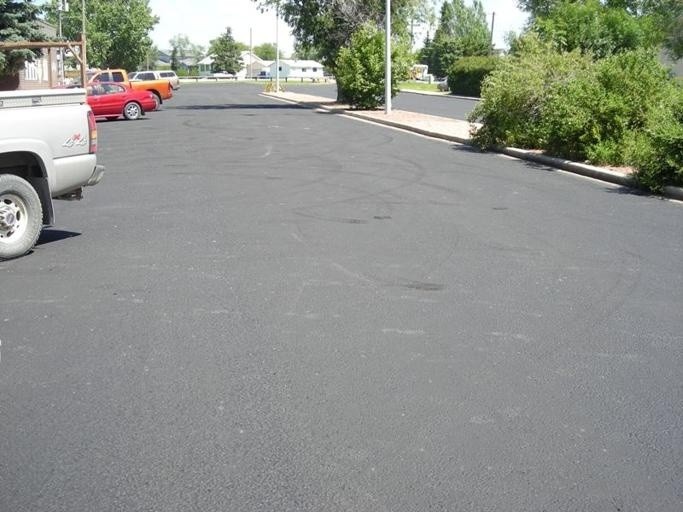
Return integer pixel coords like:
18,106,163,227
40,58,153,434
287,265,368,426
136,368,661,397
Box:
127,70,180,94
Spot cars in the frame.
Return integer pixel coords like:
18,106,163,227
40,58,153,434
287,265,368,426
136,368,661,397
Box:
436,78,451,91
55,81,155,121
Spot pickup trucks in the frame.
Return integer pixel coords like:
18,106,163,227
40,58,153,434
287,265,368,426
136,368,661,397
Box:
0,88,104,261
70,69,171,112
